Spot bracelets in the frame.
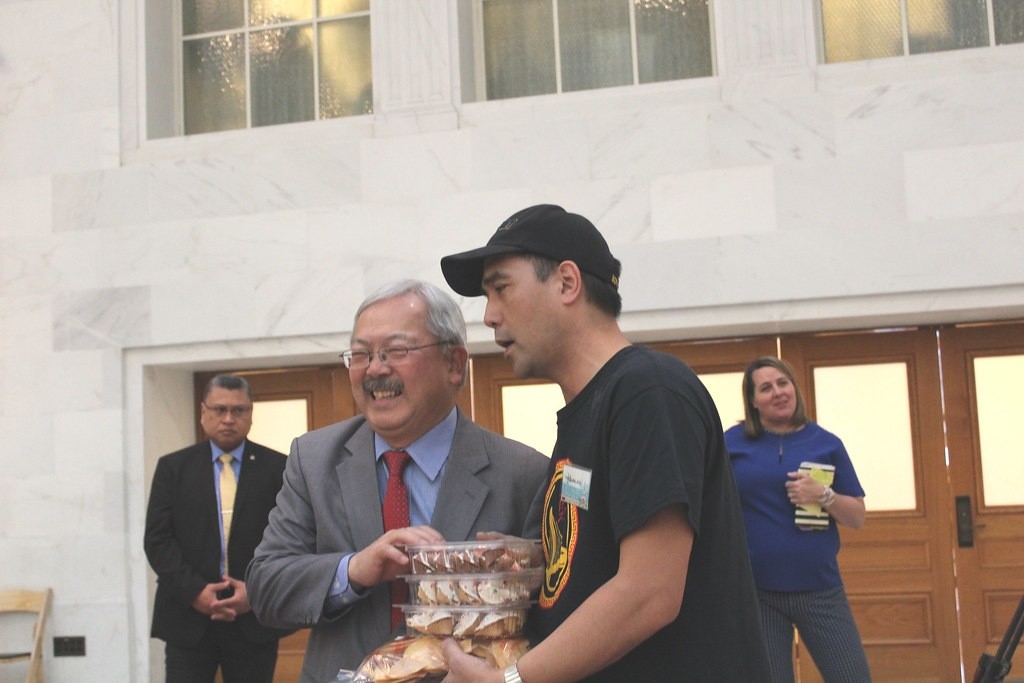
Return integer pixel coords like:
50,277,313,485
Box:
503,663,524,683
817,485,836,508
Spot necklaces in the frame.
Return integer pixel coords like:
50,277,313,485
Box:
760,422,797,462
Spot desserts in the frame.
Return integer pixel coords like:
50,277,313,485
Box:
405,541,537,637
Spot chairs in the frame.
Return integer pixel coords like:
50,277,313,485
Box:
0,589,55,682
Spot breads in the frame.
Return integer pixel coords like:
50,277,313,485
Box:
356,638,533,683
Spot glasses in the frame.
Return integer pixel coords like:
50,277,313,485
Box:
338,341,446,369
204,401,253,416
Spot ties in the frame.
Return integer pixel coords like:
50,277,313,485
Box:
382,450,413,633
220,454,238,582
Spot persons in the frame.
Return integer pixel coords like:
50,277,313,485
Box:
143,374,288,683
243,279,551,683
442,205,775,683
723,357,872,683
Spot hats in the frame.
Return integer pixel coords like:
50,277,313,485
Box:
441,203,619,297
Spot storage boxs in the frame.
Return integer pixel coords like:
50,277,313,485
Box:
392,538,544,639
